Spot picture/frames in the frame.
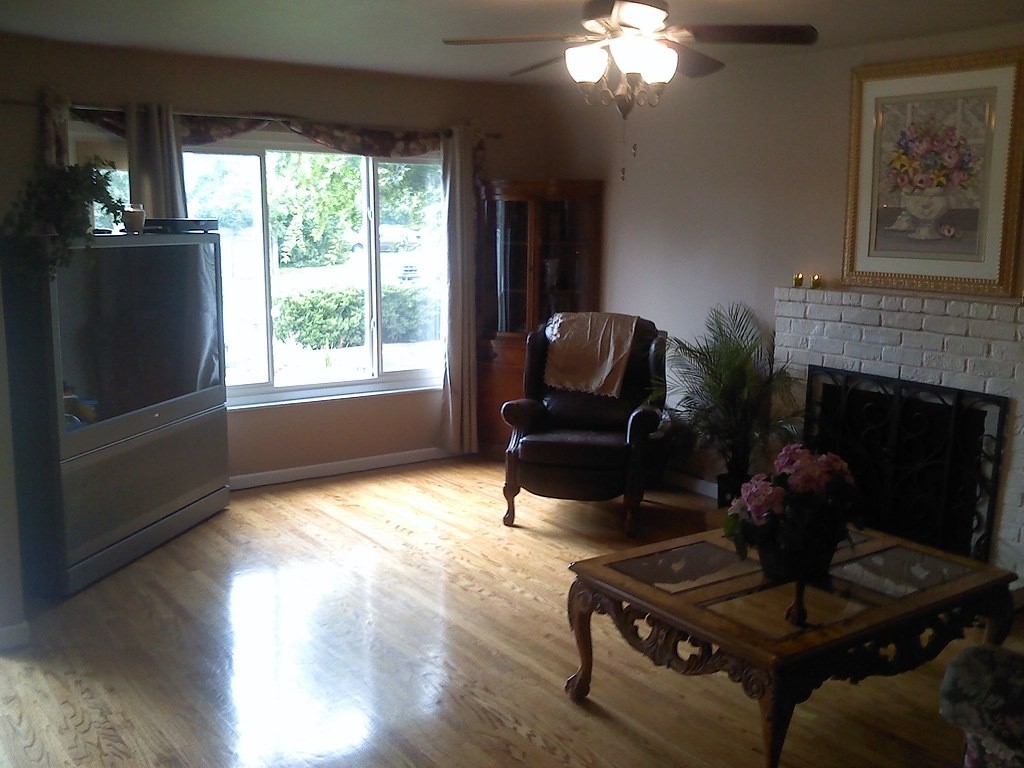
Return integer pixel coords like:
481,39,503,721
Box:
842,47,1022,301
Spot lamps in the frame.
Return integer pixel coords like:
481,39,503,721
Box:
564,2,679,183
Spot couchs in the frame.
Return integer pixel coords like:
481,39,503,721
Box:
503,310,669,536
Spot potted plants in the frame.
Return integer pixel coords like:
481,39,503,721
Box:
649,307,823,513
0,153,128,289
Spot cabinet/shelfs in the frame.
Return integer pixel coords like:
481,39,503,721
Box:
479,182,581,459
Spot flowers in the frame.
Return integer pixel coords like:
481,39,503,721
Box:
721,442,866,562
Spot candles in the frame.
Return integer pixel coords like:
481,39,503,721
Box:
792,273,803,287
810,274,820,289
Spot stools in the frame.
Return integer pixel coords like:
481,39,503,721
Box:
936,644,1024,767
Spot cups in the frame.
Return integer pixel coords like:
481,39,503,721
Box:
123,210,146,235
124,204,143,210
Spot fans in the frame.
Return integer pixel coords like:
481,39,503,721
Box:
442,1,821,82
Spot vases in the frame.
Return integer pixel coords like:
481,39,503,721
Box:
123,204,147,234
756,537,837,582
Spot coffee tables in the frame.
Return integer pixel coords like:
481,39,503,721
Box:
564,514,1021,768
